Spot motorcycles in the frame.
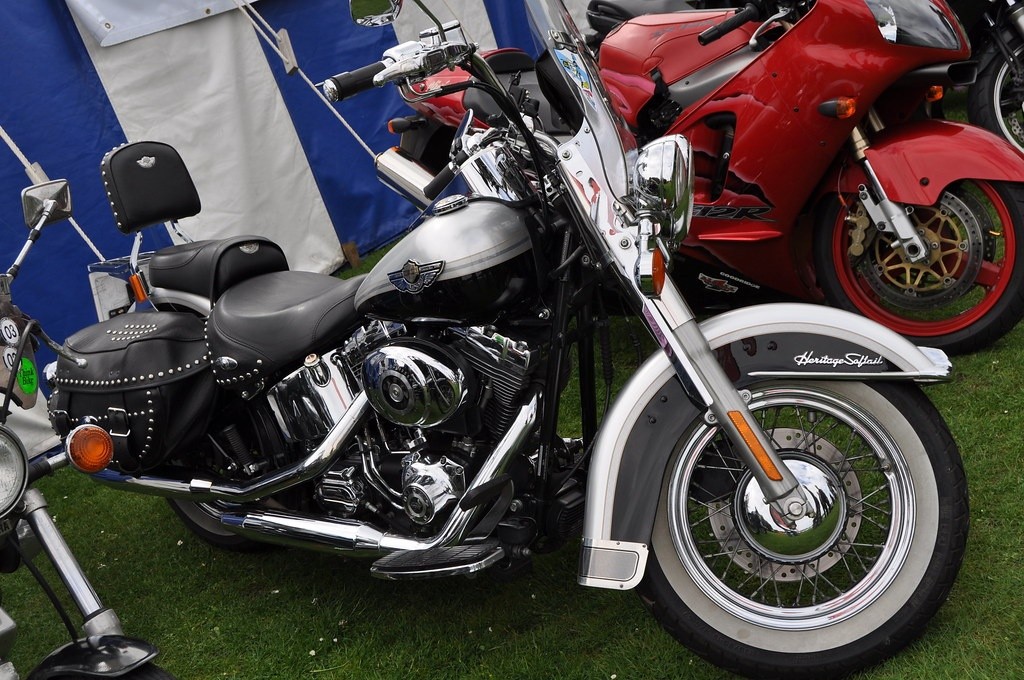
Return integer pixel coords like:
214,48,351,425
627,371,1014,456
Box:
43,1,971,676
0,175,191,679
586,2,1024,158
383,1,1024,355
